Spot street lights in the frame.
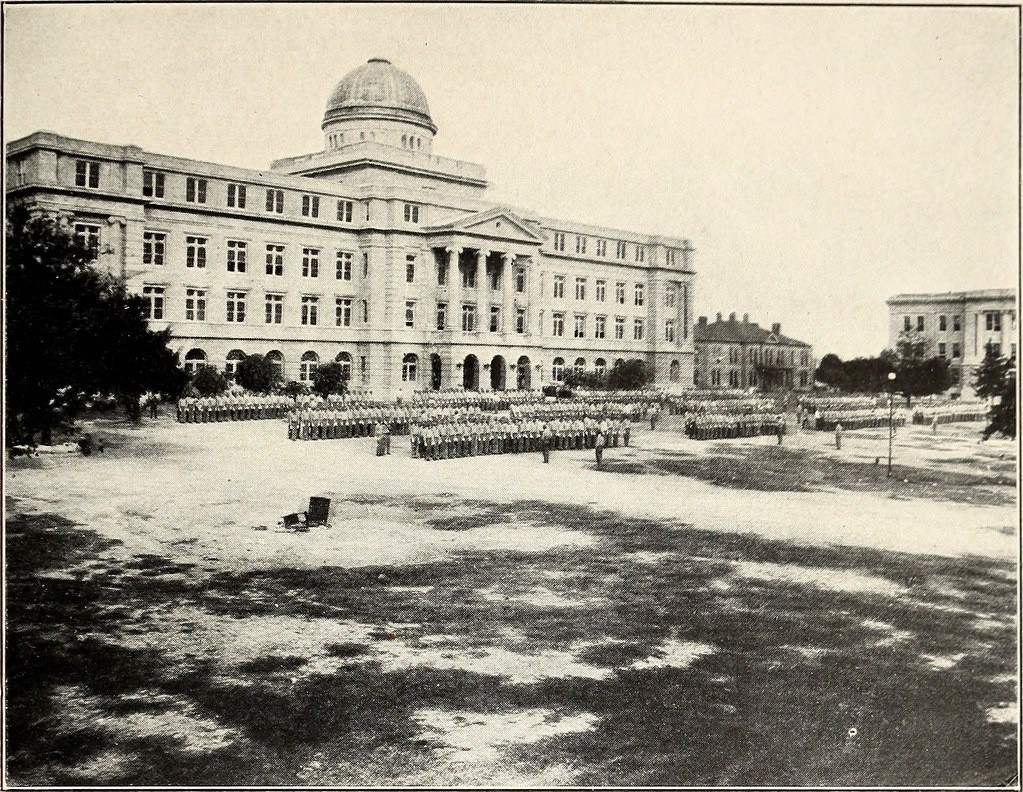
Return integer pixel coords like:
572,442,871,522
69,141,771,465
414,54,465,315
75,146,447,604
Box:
888,369,897,477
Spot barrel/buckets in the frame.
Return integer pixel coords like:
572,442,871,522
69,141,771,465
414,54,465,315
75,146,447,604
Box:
307,497,330,525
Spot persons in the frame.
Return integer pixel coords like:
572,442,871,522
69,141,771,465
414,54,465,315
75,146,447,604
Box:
146,385,994,466
